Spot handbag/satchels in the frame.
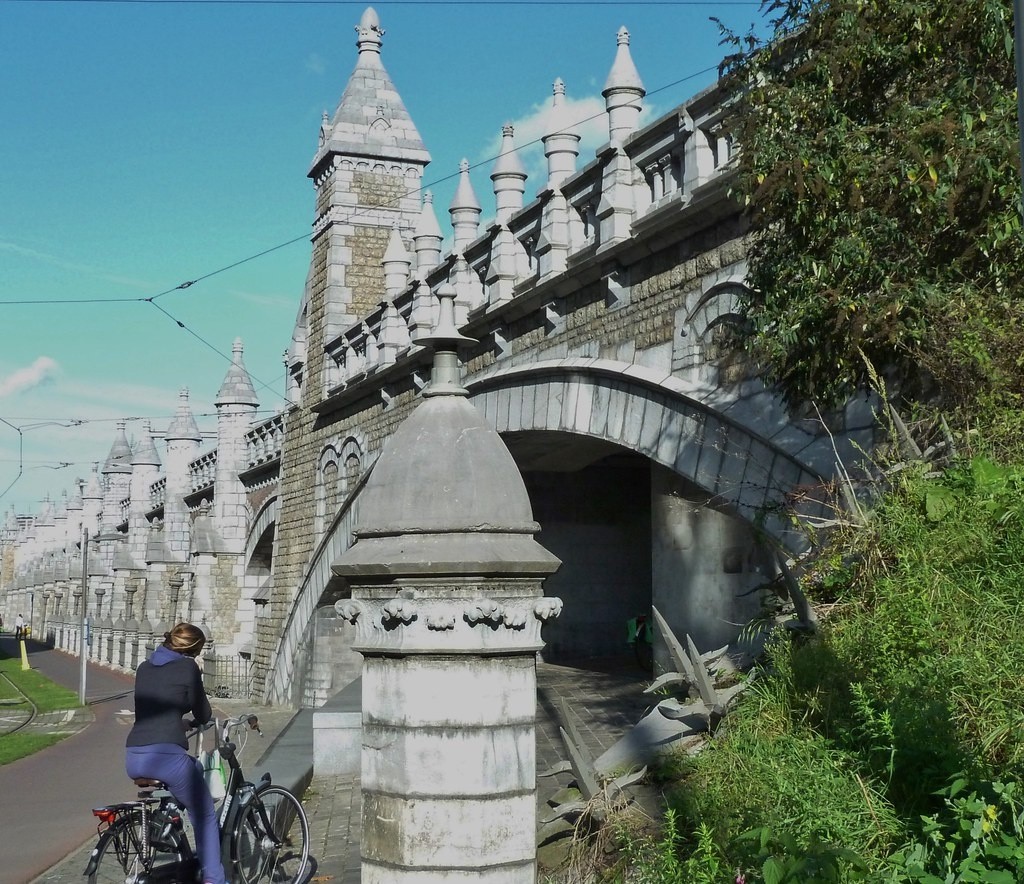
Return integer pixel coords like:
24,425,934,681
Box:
195,717,226,803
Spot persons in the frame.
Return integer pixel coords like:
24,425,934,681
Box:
0,614,3,633
16,614,23,640
126,624,230,884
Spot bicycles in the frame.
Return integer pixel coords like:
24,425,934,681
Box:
82,713,311,884
633,613,653,671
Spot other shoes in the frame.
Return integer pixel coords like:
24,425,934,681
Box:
138,826,179,853
